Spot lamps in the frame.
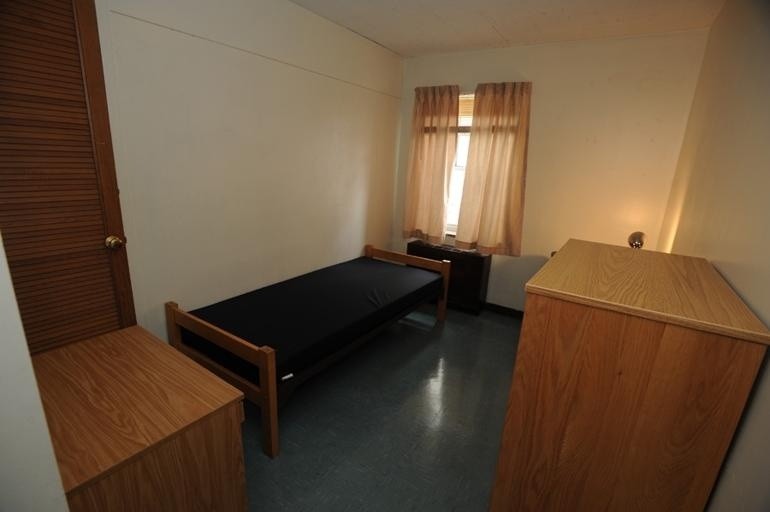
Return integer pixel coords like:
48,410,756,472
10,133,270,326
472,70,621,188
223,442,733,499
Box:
627,231,648,249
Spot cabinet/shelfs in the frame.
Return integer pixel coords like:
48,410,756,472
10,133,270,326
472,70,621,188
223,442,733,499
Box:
32,323,248,512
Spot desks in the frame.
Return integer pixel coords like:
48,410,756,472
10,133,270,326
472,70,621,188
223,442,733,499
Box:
488,239,769,512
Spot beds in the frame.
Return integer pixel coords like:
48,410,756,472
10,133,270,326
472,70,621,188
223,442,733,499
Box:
164,243,452,458
